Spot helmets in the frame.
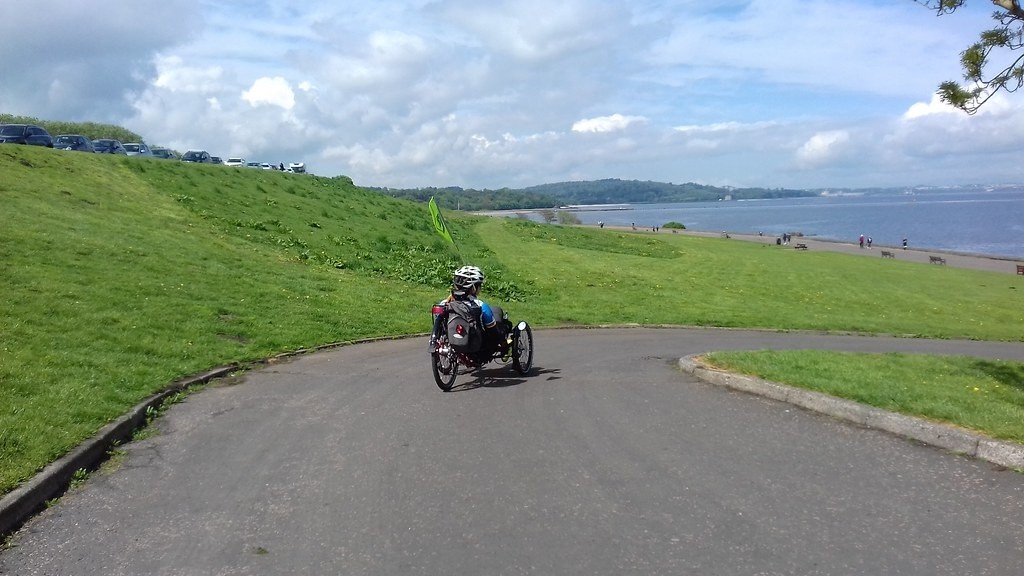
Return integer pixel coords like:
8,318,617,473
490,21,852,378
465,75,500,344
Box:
454,266,483,288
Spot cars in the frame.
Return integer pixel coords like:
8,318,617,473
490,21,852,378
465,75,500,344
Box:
0,124,53,148
54,135,95,152
122,143,152,156
93,139,127,155
209,156,222,164
151,149,177,159
247,161,306,174
225,158,245,166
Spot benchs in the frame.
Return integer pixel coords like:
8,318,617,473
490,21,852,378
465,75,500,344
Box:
881,250,894,258
1016,265,1024,275
929,256,946,265
794,243,808,249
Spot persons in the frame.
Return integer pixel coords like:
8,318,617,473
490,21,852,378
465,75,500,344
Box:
433,266,509,355
652,225,655,232
279,163,285,171
656,224,659,232
867,236,872,250
859,235,864,249
782,233,791,245
903,236,907,250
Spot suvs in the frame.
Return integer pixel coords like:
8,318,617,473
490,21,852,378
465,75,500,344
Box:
181,151,211,164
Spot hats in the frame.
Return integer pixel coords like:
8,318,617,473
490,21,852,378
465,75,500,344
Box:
861,235,863,236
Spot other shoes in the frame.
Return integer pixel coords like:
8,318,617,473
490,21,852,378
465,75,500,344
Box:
867,247,871,249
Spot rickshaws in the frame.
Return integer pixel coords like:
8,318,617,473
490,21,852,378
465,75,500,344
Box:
427,304,533,391
